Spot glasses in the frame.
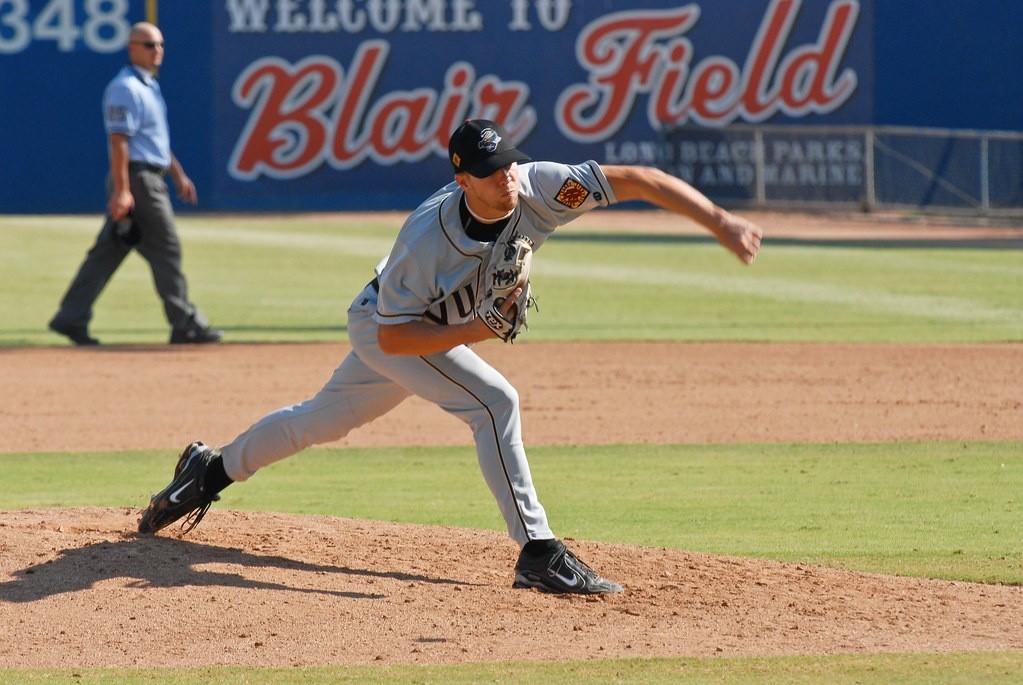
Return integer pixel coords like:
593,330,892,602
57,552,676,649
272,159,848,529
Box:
129,41,164,49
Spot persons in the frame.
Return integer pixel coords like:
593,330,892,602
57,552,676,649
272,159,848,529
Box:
48,20,223,347
137,119,767,598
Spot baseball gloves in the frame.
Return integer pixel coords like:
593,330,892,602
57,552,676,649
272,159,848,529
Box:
475,229,540,346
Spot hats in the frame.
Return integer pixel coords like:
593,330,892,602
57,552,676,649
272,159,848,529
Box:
449,119,531,178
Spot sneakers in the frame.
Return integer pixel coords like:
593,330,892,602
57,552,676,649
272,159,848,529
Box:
139,440,218,534
50,315,98,345
170,317,223,345
512,539,623,593
